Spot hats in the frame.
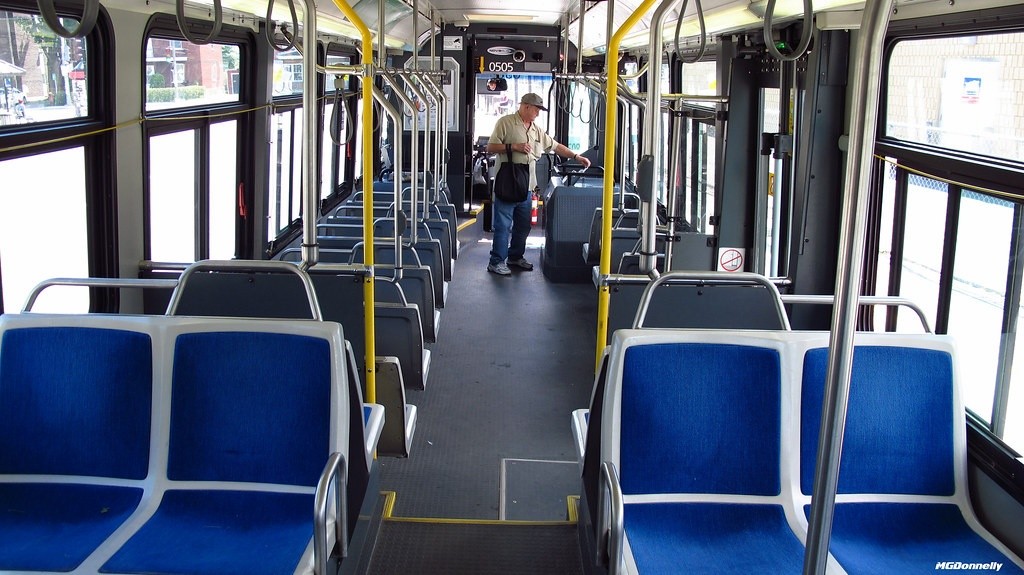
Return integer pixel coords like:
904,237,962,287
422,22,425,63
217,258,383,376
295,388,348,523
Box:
521,93,549,111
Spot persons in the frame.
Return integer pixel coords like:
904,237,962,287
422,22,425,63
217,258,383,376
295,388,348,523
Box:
487,81,500,90
488,93,591,275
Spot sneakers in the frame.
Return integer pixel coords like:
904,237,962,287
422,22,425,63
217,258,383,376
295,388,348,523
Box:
487,261,511,275
506,256,533,268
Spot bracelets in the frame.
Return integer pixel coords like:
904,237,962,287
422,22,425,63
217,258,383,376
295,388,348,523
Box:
574,154,577,159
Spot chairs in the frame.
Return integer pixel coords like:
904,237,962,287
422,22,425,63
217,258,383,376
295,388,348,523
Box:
1,169,1023,575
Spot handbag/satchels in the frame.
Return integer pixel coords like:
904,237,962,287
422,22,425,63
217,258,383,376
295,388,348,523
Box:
494,144,530,202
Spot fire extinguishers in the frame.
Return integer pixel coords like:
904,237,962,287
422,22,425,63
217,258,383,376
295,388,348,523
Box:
530,186,541,225
